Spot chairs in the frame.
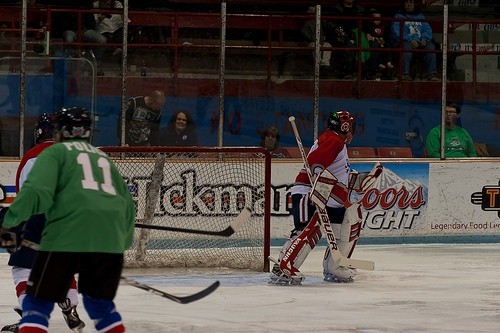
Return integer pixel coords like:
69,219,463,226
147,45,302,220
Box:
197,146,414,159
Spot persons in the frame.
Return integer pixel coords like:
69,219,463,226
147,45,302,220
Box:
425,103,477,157
117,90,165,147
302,0,456,81
268,110,362,284
0,105,136,333
160,111,200,146
93,0,131,75
251,127,294,158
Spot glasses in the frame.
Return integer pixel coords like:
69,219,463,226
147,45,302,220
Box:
445,111,456,114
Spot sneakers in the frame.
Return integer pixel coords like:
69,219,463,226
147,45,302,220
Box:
61,305,86,333
0,308,22,333
322,259,356,283
267,262,305,287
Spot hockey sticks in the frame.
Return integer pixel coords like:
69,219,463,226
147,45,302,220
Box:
289,116,375,271
134,206,255,237
21,240,220,304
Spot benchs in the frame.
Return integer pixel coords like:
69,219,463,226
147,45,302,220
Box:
0,4,442,78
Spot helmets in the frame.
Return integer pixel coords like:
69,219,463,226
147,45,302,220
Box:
52,105,92,141
33,112,54,144
327,110,355,145
